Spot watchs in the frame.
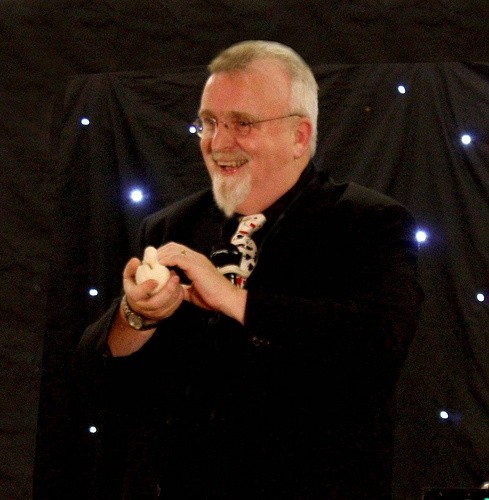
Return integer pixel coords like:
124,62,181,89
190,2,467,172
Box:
121,294,161,332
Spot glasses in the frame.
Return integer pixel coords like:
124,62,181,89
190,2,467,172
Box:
192,113,306,139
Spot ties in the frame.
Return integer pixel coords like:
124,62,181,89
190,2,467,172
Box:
231,213,266,288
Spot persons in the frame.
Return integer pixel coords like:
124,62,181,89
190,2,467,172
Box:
61,40,422,500
